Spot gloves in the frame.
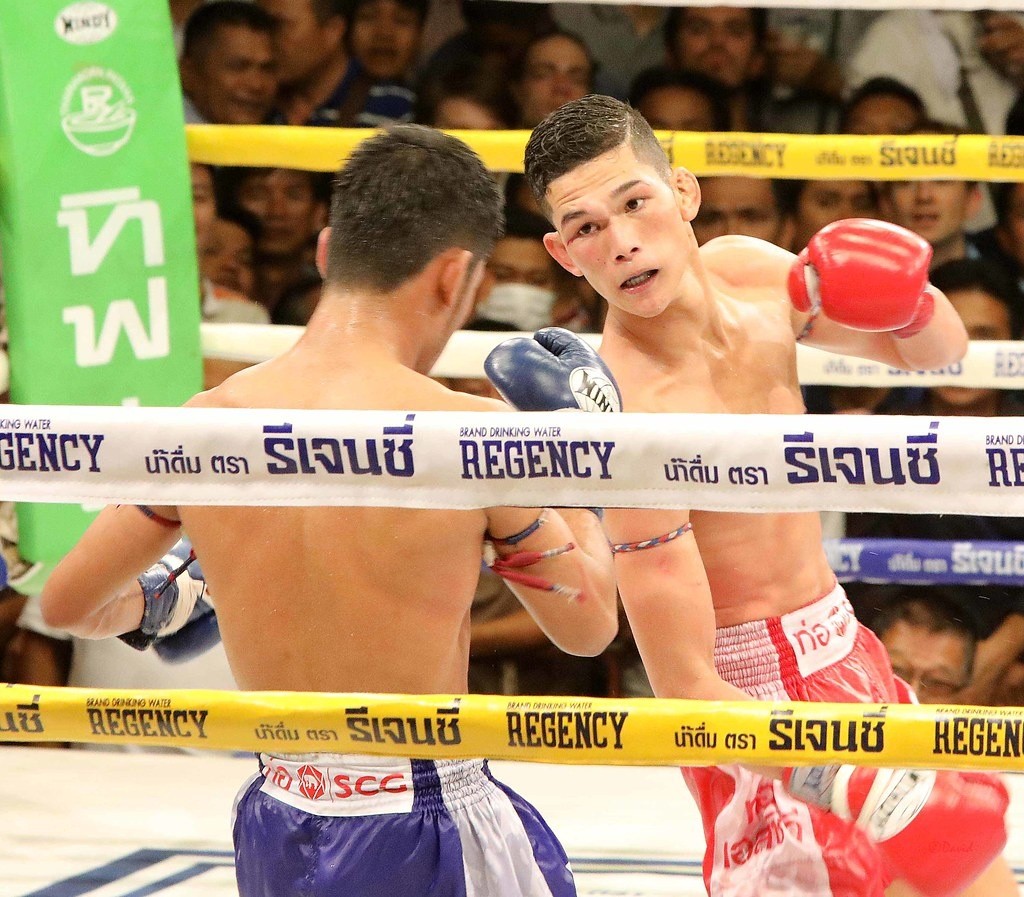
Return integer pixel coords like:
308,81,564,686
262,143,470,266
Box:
785,763,1010,896
482,326,625,518
787,215,937,337
136,536,216,636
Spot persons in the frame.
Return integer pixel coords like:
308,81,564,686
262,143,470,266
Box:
0,3,1021,714
514,95,1023,897
32,118,620,897
